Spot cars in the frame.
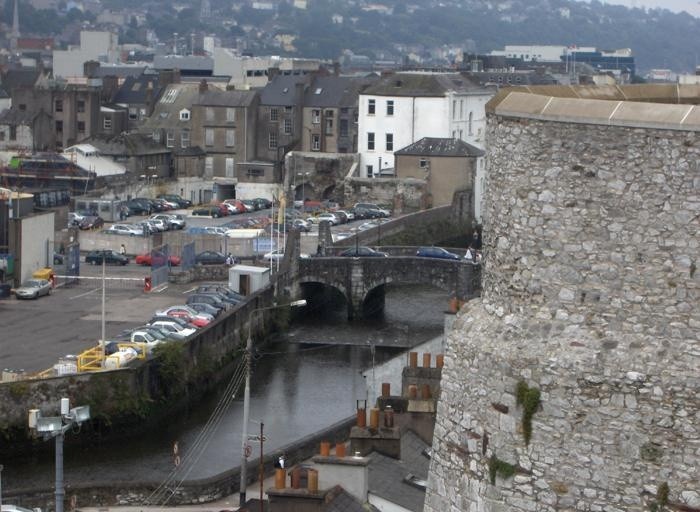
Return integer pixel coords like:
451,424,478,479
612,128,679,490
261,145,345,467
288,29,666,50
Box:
262,248,311,263
79,215,105,230
137,254,180,268
195,251,237,265
110,193,392,241
14,279,52,299
68,213,84,226
99,284,244,353
417,245,461,261
338,245,390,259
85,250,129,268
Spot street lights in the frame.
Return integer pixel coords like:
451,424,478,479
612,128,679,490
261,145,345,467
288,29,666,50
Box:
237,298,308,505
28,397,94,511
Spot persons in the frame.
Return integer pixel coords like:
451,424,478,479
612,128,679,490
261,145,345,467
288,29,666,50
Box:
476,252,482,261
315,238,326,256
225,254,231,267
119,243,126,257
462,246,473,259
277,452,285,470
229,252,234,264
